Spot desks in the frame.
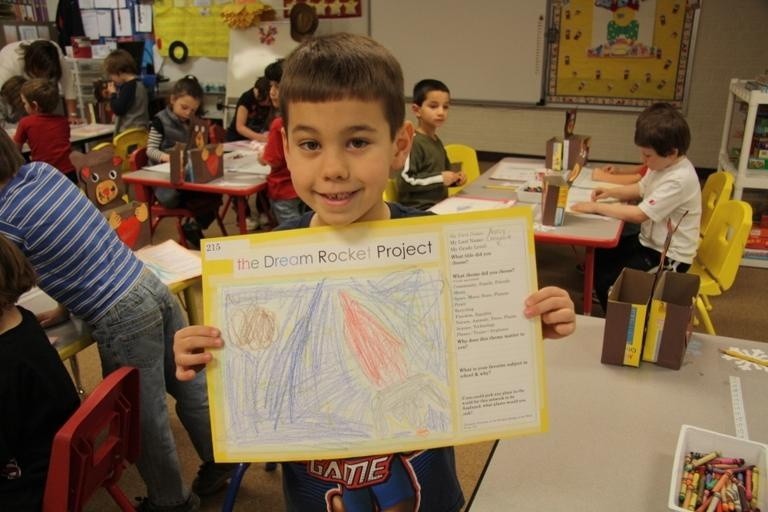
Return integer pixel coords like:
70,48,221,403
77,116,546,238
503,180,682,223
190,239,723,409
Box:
434,158,630,315
465,314,764,510
58,246,202,396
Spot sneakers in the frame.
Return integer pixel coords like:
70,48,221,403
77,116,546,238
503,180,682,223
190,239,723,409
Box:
236,216,260,231
192,460,239,493
260,213,271,228
137,498,187,512
180,216,204,247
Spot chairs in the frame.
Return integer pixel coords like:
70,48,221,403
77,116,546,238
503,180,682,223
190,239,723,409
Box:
444,144,481,198
382,178,400,203
3,121,272,245
688,201,753,335
700,170,734,311
43,367,140,512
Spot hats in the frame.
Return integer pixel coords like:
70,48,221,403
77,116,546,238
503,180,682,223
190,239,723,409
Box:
290,3,318,42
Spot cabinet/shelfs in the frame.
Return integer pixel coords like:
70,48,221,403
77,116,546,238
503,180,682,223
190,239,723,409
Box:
718,79,768,269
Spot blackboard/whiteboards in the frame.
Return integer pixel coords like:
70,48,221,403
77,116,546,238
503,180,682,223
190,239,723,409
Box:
369,0,550,106
225,18,364,108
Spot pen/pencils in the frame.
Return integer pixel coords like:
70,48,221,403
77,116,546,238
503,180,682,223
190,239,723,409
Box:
718,346,768,367
481,185,516,190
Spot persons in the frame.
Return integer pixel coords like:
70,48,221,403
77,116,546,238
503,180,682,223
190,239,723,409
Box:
569,102,703,319
0,232,83,511
146,75,224,249
0,125,251,511
104,48,160,206
171,30,576,512
1,41,79,185
227,58,301,231
395,80,468,214
